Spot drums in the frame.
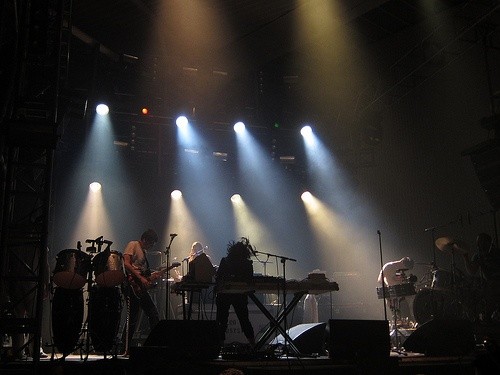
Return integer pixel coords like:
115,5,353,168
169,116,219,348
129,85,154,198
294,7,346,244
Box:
376,283,416,299
51,286,85,355
52,248,92,290
93,249,127,287
87,285,122,352
432,266,468,304
413,293,439,325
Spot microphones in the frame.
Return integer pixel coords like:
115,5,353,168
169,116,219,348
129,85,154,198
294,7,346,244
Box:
425,226,435,231
169,233,177,236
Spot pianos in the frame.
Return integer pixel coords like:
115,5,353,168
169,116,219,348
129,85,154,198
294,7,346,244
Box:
215,270,340,359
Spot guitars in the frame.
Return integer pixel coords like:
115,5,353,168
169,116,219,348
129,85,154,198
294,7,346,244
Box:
129,261,181,300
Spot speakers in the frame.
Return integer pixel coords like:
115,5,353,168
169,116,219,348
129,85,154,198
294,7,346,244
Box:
131,319,226,362
268,321,327,357
327,319,391,361
400,317,474,356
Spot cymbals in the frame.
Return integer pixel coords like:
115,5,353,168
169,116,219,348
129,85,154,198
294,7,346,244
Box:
434,237,471,255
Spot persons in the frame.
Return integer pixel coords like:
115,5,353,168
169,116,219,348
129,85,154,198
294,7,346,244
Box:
191,242,213,265
468,233,500,320
9,244,51,360
120,228,162,358
377,257,415,350
213,241,256,347
